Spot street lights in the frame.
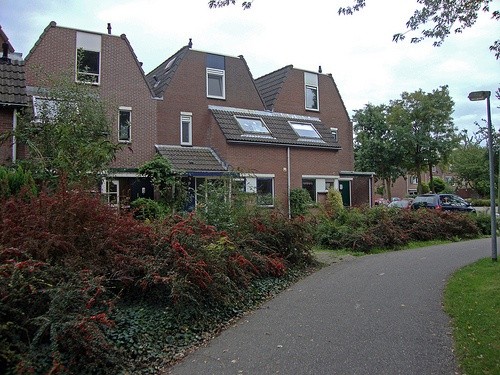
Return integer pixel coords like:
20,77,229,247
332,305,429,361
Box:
467,90,497,263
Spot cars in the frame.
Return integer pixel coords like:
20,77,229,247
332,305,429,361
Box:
374,194,414,205
387,200,411,211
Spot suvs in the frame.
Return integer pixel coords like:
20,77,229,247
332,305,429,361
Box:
409,193,477,214
